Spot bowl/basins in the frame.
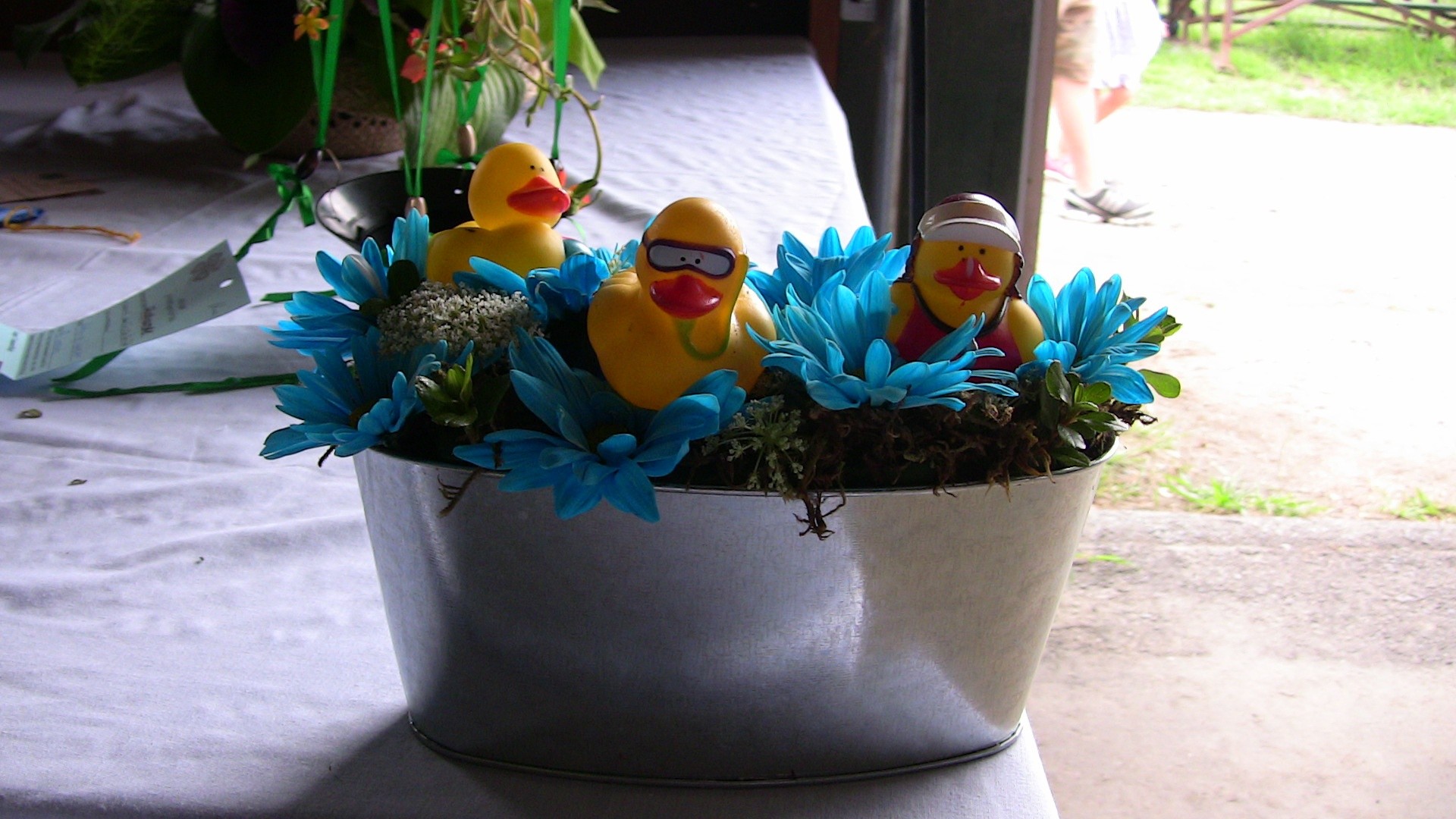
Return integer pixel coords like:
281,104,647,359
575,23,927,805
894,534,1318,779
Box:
314,167,480,266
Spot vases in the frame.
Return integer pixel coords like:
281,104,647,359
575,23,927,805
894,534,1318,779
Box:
344,412,1139,784
179,13,403,160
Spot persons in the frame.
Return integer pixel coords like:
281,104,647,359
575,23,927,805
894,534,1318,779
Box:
1043,1,1161,226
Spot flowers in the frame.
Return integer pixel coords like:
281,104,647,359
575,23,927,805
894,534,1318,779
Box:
14,0,623,218
258,204,1161,527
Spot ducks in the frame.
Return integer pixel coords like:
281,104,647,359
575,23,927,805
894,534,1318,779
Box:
588,194,777,412
425,142,571,293
889,192,1044,372
